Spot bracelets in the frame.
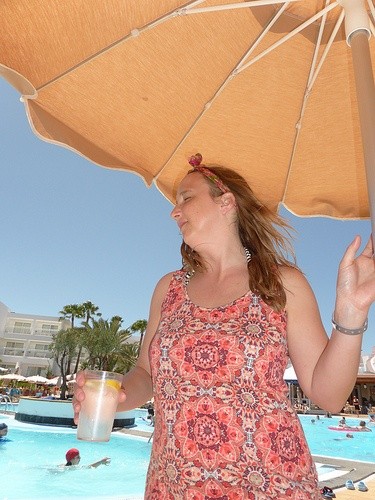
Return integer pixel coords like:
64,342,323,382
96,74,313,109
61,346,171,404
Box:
331,312,369,335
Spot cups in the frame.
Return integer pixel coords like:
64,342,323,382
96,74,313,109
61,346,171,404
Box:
76,369,123,442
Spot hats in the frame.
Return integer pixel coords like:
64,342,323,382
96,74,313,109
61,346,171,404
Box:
65,447,80,460
0,422,8,431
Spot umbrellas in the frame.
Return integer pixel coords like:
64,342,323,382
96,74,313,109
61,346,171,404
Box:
0,0,374,223
2,370,77,386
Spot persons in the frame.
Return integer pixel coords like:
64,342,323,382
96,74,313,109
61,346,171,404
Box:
339,417,345,426
2,383,64,402
352,396,360,417
0,422,9,442
359,421,371,432
68,164,373,499
346,433,353,438
61,449,112,471
325,410,331,419
369,415,375,423
